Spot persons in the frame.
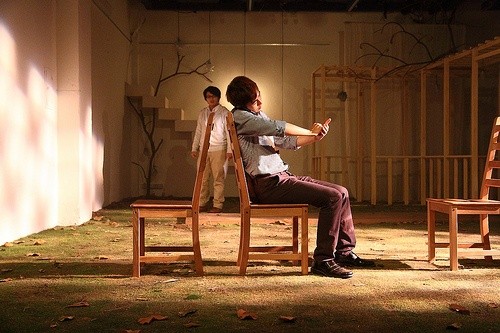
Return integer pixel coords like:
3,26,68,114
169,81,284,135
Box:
191,85,233,213
226,76,375,278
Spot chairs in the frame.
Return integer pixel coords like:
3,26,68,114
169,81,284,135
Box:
226,113,310,275
129,111,214,277
425,117,500,271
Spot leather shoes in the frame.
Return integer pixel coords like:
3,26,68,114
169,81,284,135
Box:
311,259,353,278
334,251,366,267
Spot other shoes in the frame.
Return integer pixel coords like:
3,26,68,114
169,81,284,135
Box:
199,206,207,211
208,207,223,212
